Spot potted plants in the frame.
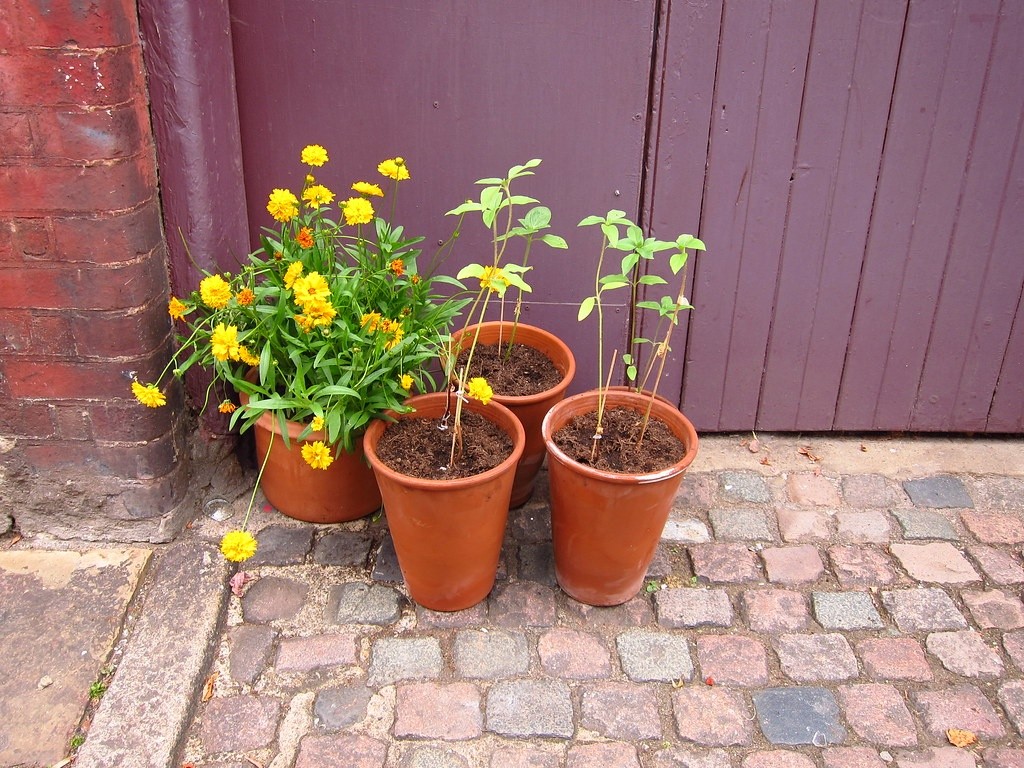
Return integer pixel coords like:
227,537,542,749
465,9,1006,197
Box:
427,207,578,507
544,209,708,607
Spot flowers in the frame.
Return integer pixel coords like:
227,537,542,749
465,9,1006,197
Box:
439,156,543,466
129,143,490,562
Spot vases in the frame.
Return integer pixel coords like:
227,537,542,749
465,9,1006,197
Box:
238,364,382,524
361,394,526,613
